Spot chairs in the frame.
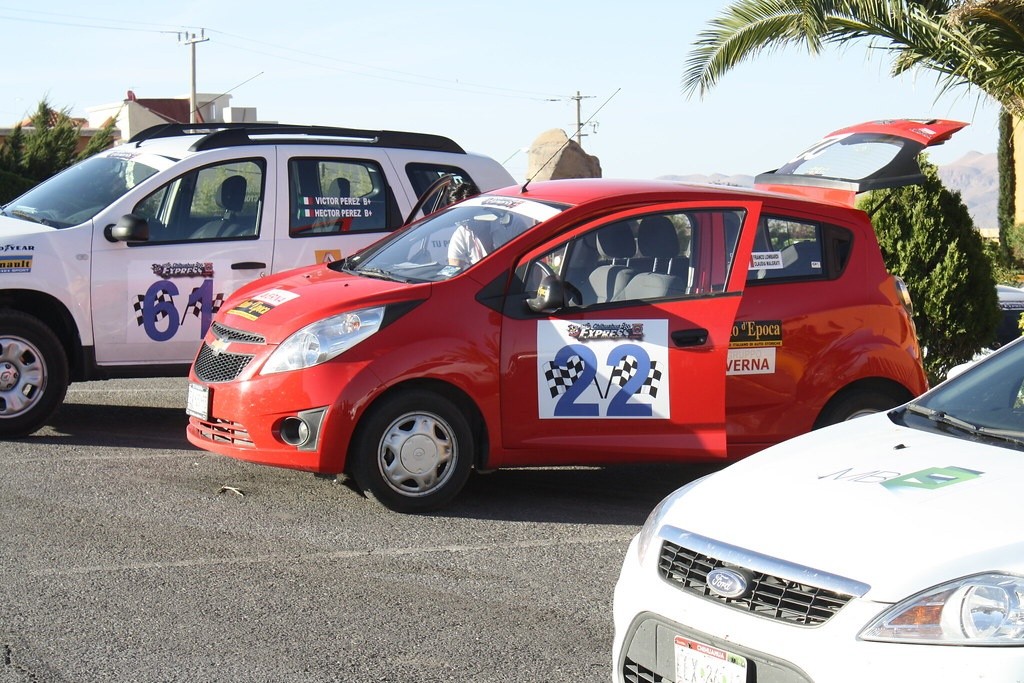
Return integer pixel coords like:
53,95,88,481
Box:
314,178,350,232
612,215,683,301
187,175,247,239
570,222,636,307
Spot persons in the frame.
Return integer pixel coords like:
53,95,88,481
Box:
448,183,542,292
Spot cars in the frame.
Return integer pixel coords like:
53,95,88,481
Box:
186,118,971,513
611,337,1024,683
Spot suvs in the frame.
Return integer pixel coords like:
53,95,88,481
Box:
0,123,536,439
